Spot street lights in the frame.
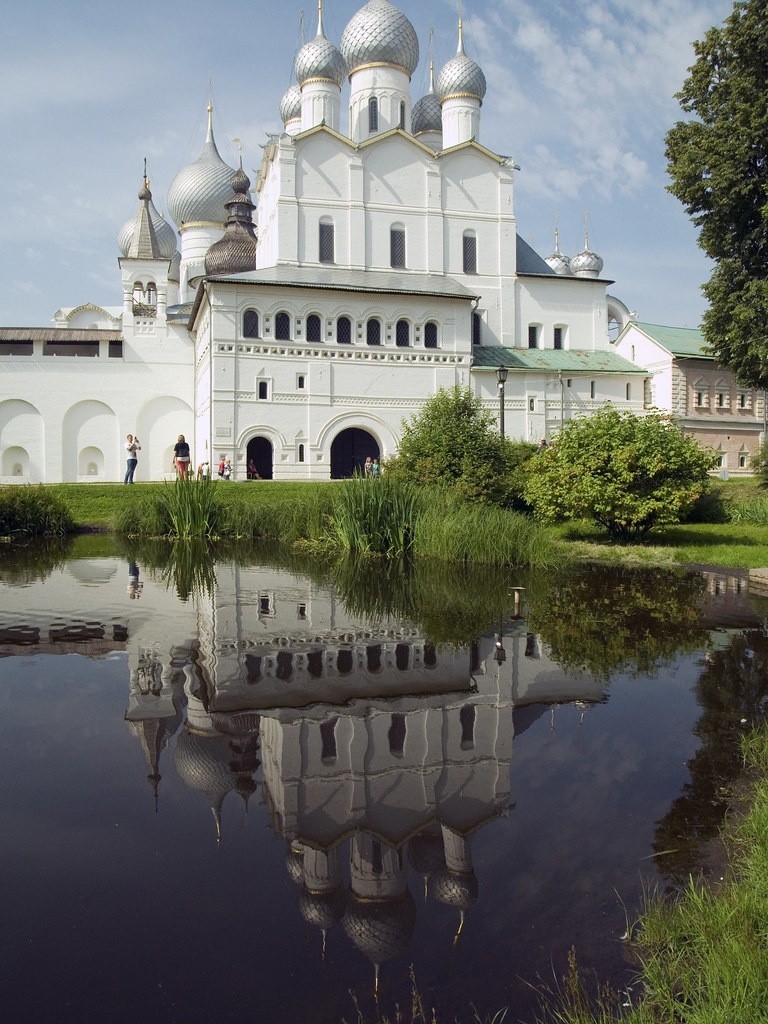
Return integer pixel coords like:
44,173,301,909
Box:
493,617,506,666
495,364,509,445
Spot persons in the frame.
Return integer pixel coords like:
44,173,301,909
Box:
373,459,379,479
248,459,262,480
125,434,141,485
218,456,225,479
223,460,232,480
174,435,191,482
202,461,209,480
364,457,373,480
198,462,204,480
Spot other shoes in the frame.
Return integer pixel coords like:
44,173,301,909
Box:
130,482,134,484
124,480,127,485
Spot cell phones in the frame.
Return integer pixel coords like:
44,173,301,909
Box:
135,436,136,439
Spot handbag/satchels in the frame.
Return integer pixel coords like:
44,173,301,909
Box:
187,462,194,476
223,469,231,476
218,471,224,476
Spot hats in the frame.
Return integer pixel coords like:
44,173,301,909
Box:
204,461,209,464
